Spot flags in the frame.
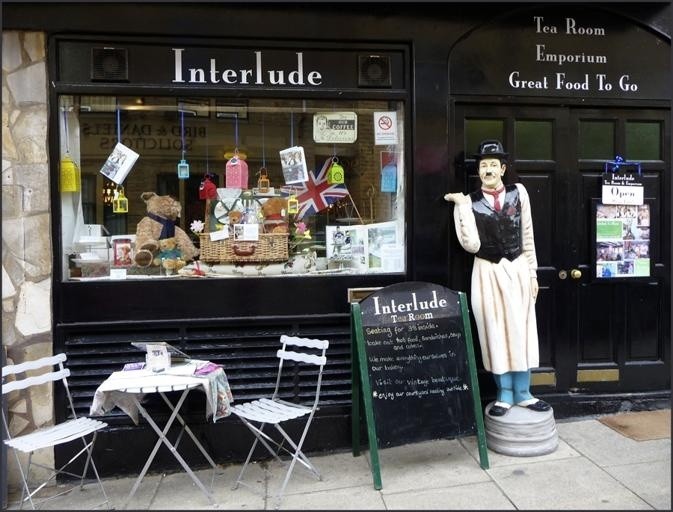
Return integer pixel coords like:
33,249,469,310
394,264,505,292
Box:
280,156,348,224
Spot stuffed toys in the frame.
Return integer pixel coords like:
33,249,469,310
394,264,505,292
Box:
228,211,242,235
260,197,289,234
131,190,201,275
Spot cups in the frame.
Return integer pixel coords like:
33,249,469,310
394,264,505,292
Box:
145,353,172,375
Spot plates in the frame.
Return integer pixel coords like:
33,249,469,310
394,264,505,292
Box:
213,199,244,224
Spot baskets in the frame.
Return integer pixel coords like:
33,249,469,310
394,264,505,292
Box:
199,200,289,262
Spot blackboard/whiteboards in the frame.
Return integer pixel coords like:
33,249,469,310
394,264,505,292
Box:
350,282,483,450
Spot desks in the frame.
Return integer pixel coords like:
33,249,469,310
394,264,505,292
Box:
88,357,234,509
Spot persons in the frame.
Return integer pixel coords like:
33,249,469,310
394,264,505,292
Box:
101,152,126,179
345,231,351,245
443,139,551,416
281,152,304,181
314,116,327,142
334,226,344,245
601,204,650,277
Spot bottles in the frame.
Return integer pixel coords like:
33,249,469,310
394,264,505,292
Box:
245,208,253,224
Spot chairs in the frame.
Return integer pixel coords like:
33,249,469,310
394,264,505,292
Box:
227,332,331,511
0,350,111,511
